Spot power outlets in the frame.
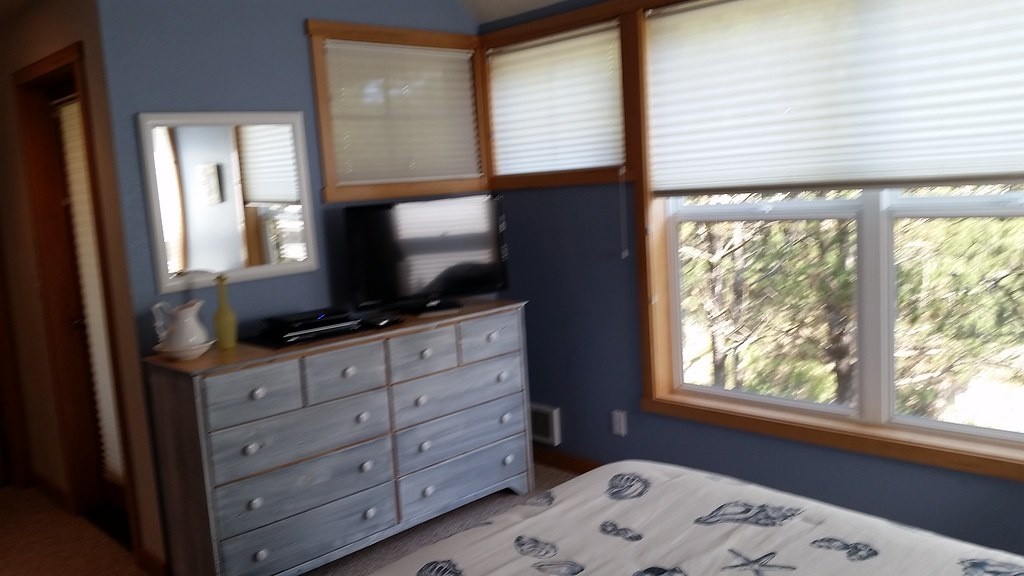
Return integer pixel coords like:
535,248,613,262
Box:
612,410,628,437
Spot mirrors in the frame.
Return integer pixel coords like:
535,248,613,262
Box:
134,111,319,295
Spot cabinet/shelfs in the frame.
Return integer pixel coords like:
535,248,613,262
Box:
145,297,538,576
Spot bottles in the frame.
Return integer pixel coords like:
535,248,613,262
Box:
211,274,236,351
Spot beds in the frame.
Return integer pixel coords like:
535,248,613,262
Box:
373,459,1024,576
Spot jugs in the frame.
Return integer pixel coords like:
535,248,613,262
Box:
149,298,208,348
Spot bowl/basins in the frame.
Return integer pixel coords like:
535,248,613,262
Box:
152,340,217,360
